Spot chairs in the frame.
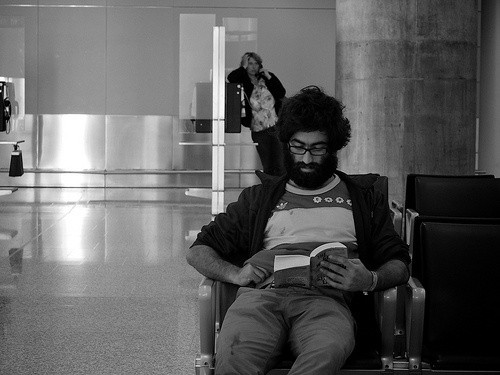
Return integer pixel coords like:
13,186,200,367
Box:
195,174,500,375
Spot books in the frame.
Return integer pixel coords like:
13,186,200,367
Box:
273,241,349,289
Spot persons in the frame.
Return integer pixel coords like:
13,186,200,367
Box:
184,85,412,375
227,52,290,176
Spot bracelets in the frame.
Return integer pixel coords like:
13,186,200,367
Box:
363,270,378,296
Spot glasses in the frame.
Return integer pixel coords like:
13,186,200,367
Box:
288,142,329,156
